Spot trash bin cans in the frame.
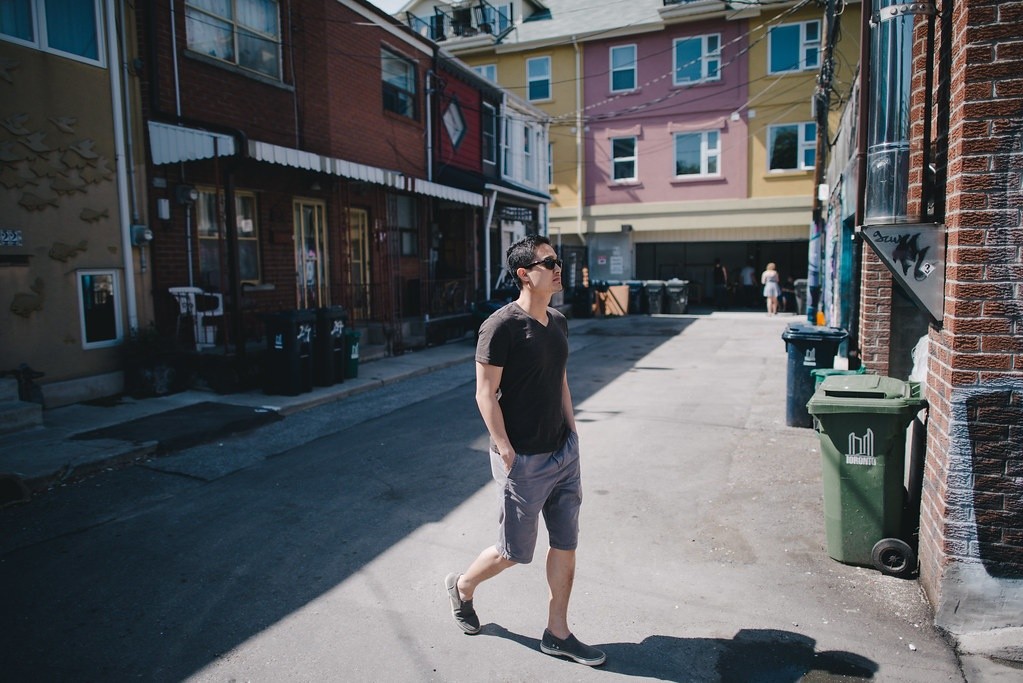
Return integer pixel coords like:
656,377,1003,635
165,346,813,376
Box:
474,299,508,346
794,279,807,315
266,305,361,395
593,278,691,314
805,368,927,577
782,320,849,429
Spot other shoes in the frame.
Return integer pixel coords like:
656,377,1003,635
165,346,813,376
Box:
540,627,606,666
446,572,481,635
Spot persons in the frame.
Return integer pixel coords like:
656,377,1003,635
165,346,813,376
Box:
445,234,606,666
714,259,796,315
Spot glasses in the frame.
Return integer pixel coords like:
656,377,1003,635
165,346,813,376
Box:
524,257,562,270
